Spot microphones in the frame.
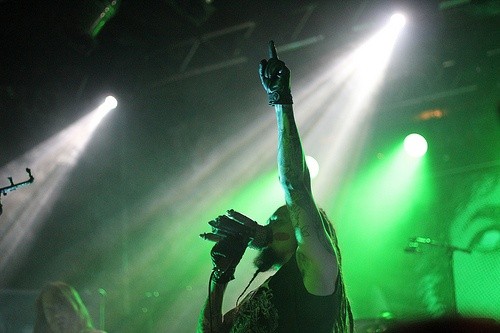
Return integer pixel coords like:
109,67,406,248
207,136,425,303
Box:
199,210,273,249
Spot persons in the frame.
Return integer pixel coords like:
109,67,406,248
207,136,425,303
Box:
192,38,357,333
32,279,109,333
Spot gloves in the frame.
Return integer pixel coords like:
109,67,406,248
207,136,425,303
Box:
210,236,249,282
259,41,294,106
